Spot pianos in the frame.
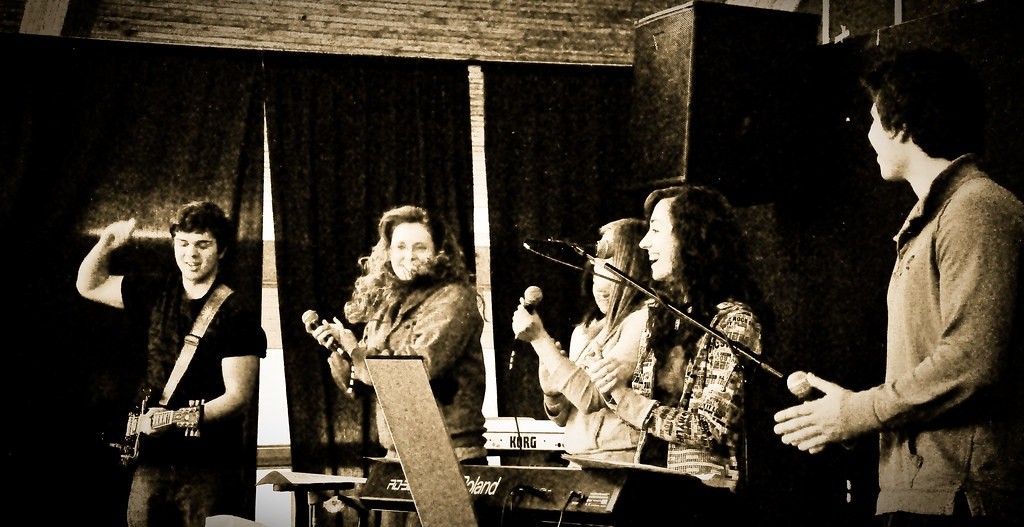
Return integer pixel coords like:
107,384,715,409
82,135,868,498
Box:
360,461,707,527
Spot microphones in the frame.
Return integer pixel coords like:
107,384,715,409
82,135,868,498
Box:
509,286,542,370
301,310,351,363
787,371,862,450
524,237,615,260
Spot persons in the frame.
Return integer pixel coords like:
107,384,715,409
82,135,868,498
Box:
775,40,1023,527
76,200,268,527
305,204,488,527
512,185,763,527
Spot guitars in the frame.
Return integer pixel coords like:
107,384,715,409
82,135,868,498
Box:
100,398,203,467
